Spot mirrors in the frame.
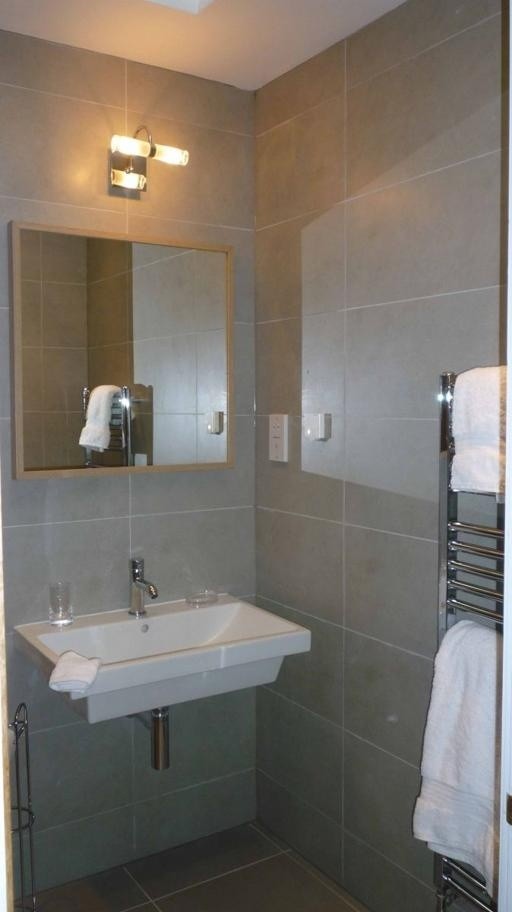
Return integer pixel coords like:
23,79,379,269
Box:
11,222,239,480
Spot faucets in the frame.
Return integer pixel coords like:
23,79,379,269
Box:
123,554,159,615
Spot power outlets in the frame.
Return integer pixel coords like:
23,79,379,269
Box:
267,412,290,463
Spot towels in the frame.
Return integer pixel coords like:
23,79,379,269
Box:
78,384,123,454
48,651,100,700
449,362,503,497
409,621,502,900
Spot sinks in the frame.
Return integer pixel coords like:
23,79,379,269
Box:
15,600,310,726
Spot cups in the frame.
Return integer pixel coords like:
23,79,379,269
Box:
183,564,218,608
46,581,75,628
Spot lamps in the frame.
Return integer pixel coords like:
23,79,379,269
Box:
107,123,190,194
